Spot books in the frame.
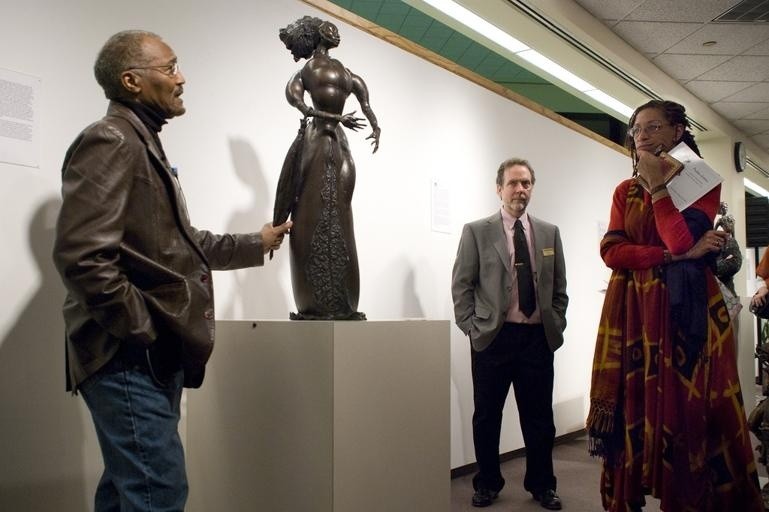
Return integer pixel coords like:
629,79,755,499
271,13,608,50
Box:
635,151,685,196
657,140,725,216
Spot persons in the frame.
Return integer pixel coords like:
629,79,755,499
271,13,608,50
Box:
751,242,769,307
268,15,381,319
451,157,569,509
51,29,294,512
715,200,742,297
585,100,767,511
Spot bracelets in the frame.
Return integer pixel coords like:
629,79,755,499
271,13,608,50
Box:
651,185,666,195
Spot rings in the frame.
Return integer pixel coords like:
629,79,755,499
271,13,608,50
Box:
716,236,720,243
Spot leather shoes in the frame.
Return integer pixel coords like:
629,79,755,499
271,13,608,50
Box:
473,477,505,507
533,489,561,510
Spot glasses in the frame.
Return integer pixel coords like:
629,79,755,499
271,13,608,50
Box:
129,61,179,76
630,119,672,136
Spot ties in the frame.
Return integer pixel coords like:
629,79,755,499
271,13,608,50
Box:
513,220,536,318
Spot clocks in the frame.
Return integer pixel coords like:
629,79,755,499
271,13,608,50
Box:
734,142,747,173
